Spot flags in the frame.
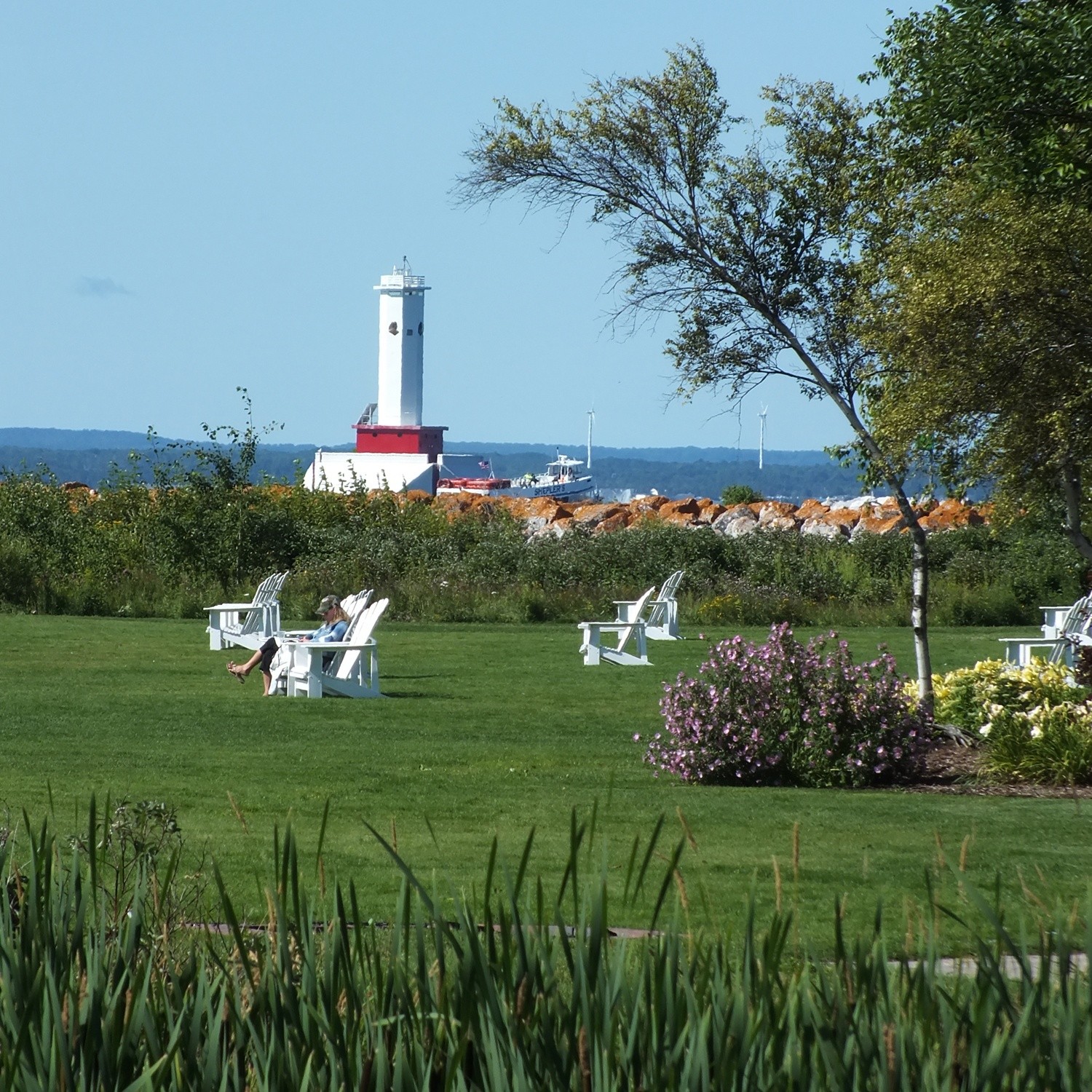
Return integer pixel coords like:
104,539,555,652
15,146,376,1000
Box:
477,461,489,470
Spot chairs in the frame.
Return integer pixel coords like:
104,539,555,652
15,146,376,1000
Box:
202,571,389,701
997,592,1092,670
579,570,692,667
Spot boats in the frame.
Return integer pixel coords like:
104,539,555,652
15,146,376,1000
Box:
434,454,596,505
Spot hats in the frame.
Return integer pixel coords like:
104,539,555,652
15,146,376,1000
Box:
314,595,340,616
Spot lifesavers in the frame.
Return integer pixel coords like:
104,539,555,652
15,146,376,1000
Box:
436,478,450,488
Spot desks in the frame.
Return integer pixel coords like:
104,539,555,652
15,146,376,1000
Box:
1038,607,1073,639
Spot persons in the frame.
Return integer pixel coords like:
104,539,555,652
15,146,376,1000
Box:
226,595,352,696
521,472,539,489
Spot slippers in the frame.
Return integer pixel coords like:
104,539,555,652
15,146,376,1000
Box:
226,663,245,683
